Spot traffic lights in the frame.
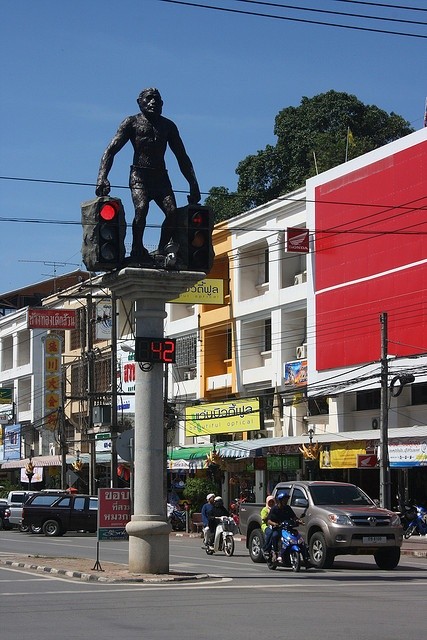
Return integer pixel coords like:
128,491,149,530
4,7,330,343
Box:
81,199,128,270
176,204,216,271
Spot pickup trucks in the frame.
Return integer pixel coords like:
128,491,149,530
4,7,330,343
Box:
4,490,71,532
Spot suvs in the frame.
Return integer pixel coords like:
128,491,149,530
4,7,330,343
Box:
23,493,98,536
239,479,404,572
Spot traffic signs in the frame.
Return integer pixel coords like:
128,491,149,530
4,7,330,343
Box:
97,487,133,542
117,428,135,462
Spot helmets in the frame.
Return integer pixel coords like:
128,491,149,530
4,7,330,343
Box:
277,492,290,501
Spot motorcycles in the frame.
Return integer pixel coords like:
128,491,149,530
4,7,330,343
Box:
201,515,235,557
266,513,306,571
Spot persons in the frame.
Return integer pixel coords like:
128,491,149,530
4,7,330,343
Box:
94,86,202,266
202,492,231,547
261,493,309,563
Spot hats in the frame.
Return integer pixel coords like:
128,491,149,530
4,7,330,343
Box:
206,493,215,502
214,496,222,502
